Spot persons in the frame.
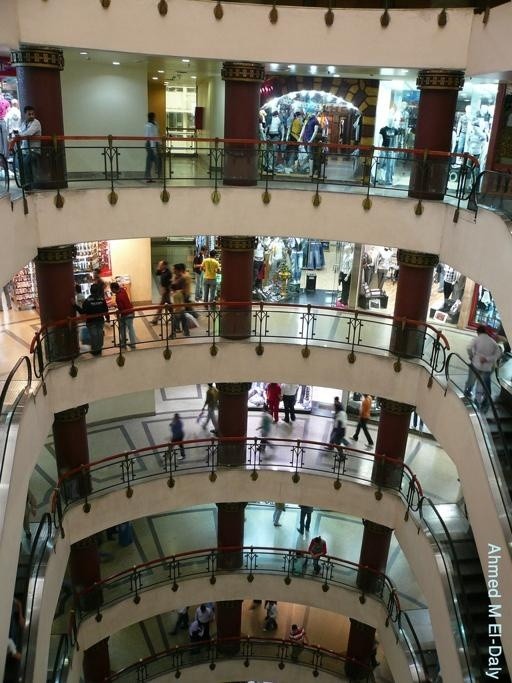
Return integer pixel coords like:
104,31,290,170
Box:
259,100,494,186
291,552,308,576
8,639,22,673
107,525,119,540
442,263,459,309
256,238,393,303
475,330,506,405
161,381,375,457
24,496,38,539
271,498,286,527
463,322,501,412
0,94,12,160
308,537,326,576
142,111,166,181
72,246,223,356
296,503,314,535
5,98,22,155
171,599,308,664
12,599,27,628
12,106,44,194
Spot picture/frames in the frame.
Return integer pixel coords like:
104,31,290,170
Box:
433,311,449,323
368,299,380,309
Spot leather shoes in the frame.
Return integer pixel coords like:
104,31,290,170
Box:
282,418,289,423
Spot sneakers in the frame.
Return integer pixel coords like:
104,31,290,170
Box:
144,180,168,183
349,437,358,442
324,446,334,451
297,523,309,534
148,320,158,325
178,456,185,460
366,443,375,447
127,343,135,348
274,522,282,526
346,443,352,448
